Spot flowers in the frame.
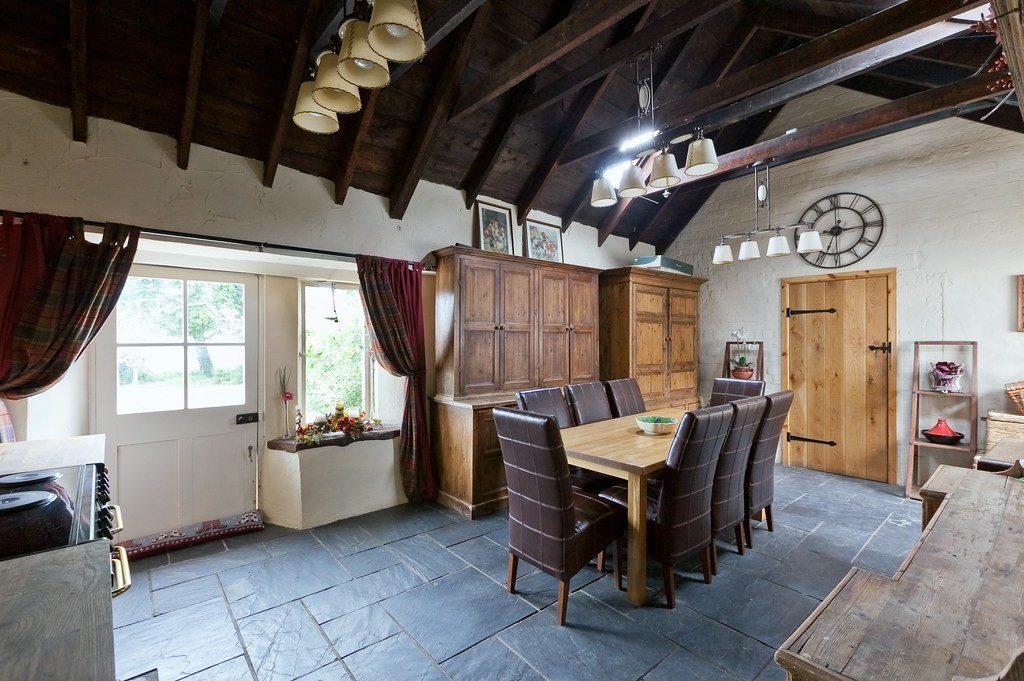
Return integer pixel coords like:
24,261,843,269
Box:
729,328,755,366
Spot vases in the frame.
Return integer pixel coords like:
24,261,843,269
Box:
731,366,754,380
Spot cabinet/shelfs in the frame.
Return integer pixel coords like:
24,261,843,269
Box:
599,265,709,403
905,339,978,501
645,394,705,411
431,241,538,401
539,258,606,389
429,393,519,522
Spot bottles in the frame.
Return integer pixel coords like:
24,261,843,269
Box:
295,405,302,439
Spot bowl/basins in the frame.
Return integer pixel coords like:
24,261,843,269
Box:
635,415,678,434
921,429,965,444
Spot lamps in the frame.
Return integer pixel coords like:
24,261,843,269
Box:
293,58,340,135
712,156,823,265
335,5,391,89
313,34,363,114
367,1,427,63
590,44,720,208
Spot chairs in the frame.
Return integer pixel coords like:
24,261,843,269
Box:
565,381,613,426
514,385,609,494
710,395,767,576
601,377,647,418
745,390,795,549
493,407,628,626
597,402,736,609
710,377,766,407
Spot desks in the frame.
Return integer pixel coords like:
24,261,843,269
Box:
774,463,1024,681
972,449,1024,479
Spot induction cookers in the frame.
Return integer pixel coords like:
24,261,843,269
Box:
0,462,114,567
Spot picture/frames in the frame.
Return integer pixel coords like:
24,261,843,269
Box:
475,199,515,255
522,218,564,264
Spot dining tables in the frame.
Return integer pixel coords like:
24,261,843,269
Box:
560,408,692,607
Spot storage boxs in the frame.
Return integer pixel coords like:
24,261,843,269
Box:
985,409,1024,462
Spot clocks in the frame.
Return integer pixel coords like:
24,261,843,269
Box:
794,192,884,269
